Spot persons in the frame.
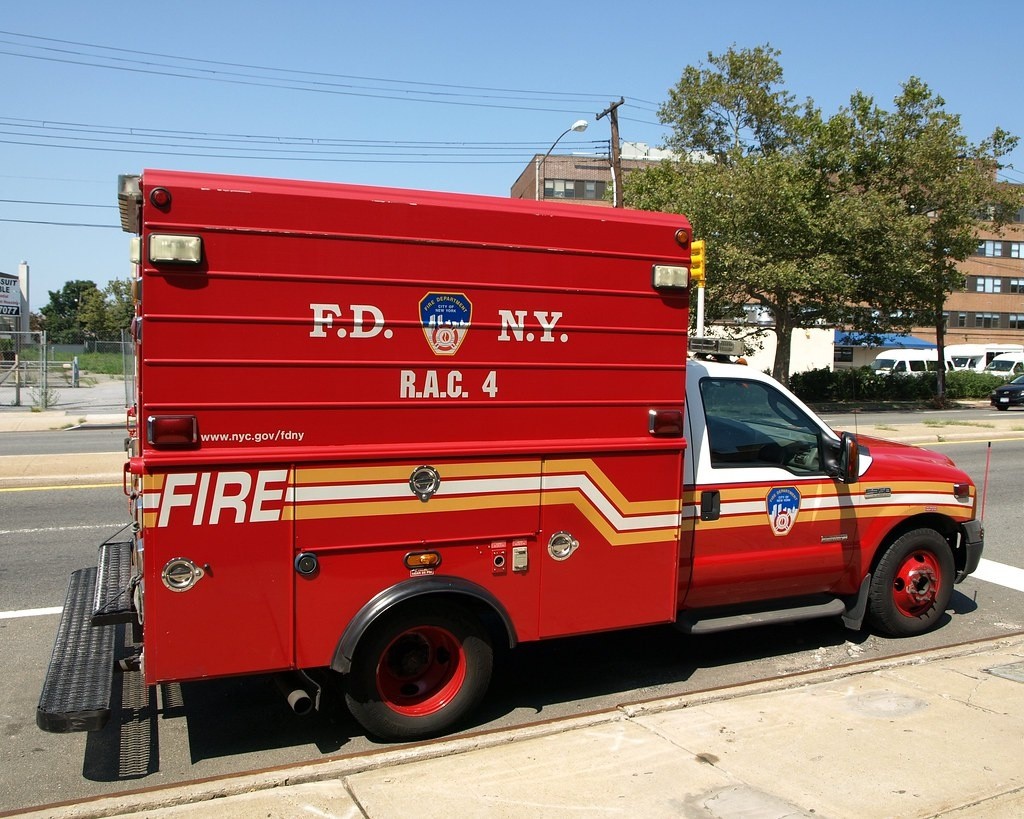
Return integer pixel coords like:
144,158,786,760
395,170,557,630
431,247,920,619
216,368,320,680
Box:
1016,363,1022,370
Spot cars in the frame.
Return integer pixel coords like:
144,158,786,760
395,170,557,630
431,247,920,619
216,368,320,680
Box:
990,376,1024,410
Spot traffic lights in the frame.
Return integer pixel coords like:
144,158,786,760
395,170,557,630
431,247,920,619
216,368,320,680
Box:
690,241,705,281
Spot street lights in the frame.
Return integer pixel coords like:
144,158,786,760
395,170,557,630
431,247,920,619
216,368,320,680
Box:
536,120,587,200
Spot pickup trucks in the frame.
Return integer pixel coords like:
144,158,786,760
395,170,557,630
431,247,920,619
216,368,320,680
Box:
37,168,993,736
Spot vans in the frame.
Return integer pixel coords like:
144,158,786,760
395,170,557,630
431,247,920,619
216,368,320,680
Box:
947,344,1024,377
870,348,955,377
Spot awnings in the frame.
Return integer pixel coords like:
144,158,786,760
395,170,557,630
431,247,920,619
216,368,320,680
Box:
835,330,938,349
